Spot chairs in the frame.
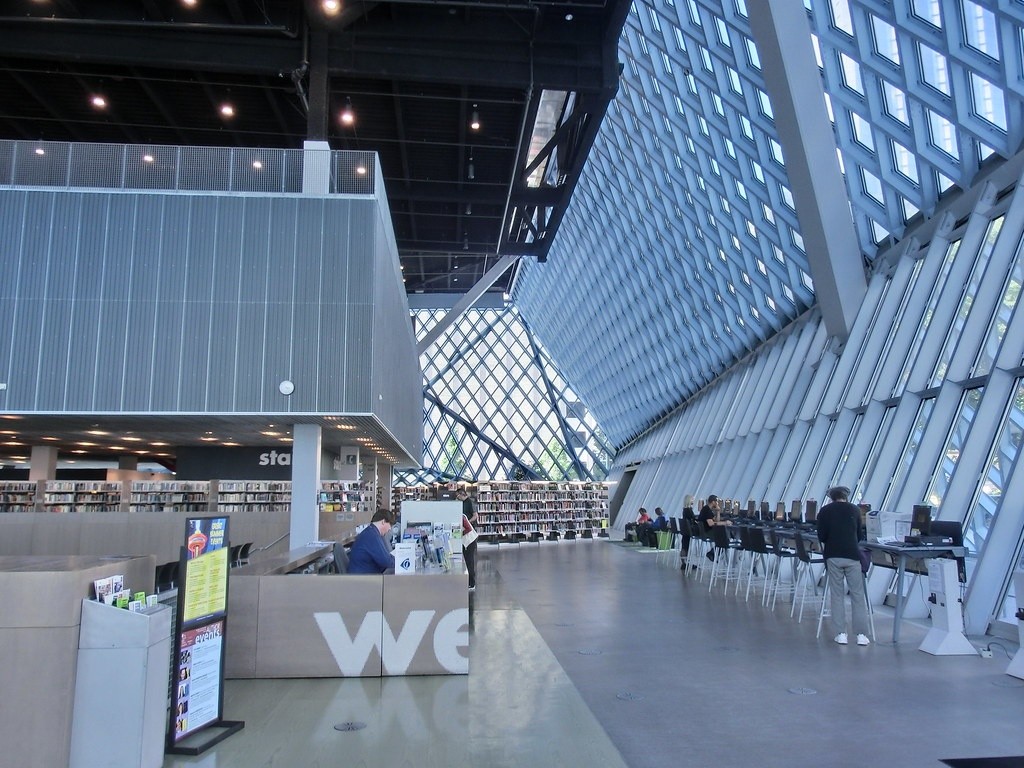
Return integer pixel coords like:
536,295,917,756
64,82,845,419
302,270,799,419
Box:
164,559,181,591
229,545,243,570
155,564,170,594
238,541,254,568
655,516,876,642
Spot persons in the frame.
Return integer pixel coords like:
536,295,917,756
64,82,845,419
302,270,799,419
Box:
180,668,189,682
177,702,184,717
463,515,477,589
698,495,731,563
346,509,396,574
623,508,650,542
181,650,190,665
649,507,667,547
680,495,697,570
816,486,870,646
179,686,185,699
176,719,181,733
454,488,477,532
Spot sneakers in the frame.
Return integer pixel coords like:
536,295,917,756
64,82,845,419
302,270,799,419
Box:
857,633,870,645
834,632,848,644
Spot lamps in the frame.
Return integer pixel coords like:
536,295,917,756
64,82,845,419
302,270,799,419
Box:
466,204,473,215
470,111,480,129
467,165,476,181
355,158,366,175
453,276,458,281
453,260,459,269
343,103,355,123
463,240,469,250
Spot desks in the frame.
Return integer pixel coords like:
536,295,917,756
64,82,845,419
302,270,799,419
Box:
732,523,970,644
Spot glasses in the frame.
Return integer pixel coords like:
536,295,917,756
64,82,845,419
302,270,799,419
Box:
456,494,460,498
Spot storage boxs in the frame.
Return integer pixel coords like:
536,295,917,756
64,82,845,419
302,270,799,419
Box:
392,543,421,573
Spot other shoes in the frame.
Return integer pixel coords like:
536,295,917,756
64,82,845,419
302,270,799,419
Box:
681,563,697,570
469,586,475,589
706,551,718,563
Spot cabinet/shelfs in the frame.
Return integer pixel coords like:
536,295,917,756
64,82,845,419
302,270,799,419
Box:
0,480,611,550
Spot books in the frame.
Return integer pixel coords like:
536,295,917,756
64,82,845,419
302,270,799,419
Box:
395,487,429,516
403,522,461,567
218,483,292,513
477,482,609,534
45,480,122,512
130,481,209,512
320,482,365,511
0,481,37,512
93,575,157,613
